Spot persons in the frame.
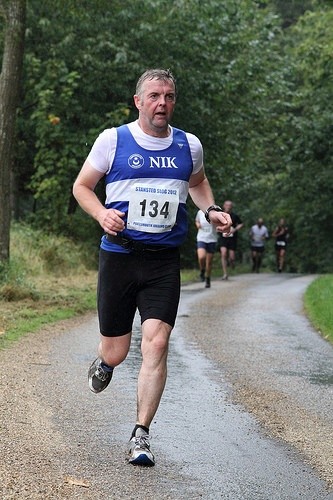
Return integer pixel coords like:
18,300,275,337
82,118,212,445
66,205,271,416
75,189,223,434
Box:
248,218,269,274
219,200,244,280
195,209,218,288
272,218,292,273
73,68,233,467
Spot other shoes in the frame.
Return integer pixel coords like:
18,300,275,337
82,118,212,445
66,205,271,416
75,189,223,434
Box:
206,277,210,287
222,274,228,280
230,262,234,267
200,273,205,281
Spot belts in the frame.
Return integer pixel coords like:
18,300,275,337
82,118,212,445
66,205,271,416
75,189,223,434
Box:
107,233,177,258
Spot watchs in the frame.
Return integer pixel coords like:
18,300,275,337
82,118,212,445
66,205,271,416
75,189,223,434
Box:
205,205,223,224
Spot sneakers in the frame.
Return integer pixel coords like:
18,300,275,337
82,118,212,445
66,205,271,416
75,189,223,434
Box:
128,428,155,467
88,357,114,394
